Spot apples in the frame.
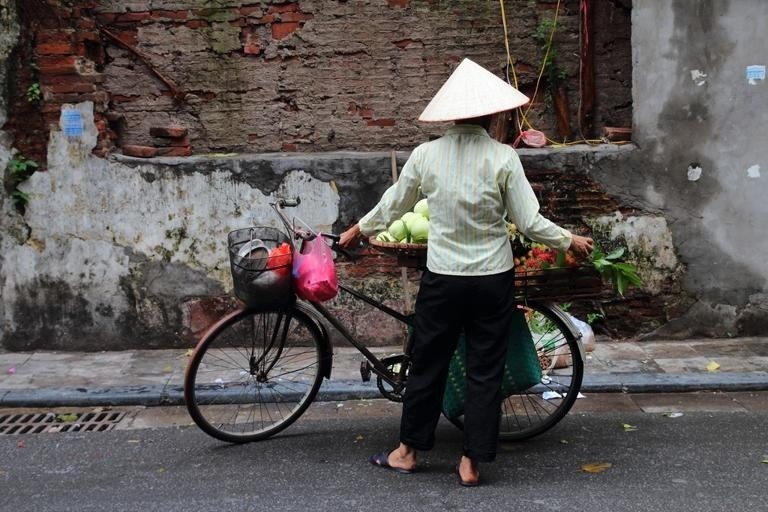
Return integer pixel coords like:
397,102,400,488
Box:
514,250,576,288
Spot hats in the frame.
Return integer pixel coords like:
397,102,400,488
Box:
418,58,530,122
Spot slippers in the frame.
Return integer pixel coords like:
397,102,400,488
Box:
368,449,412,474
455,463,480,487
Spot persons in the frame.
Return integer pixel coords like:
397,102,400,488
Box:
338,59,594,486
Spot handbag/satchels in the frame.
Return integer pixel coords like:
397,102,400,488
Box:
408,307,542,419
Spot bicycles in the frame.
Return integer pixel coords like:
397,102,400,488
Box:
184,199,601,442
